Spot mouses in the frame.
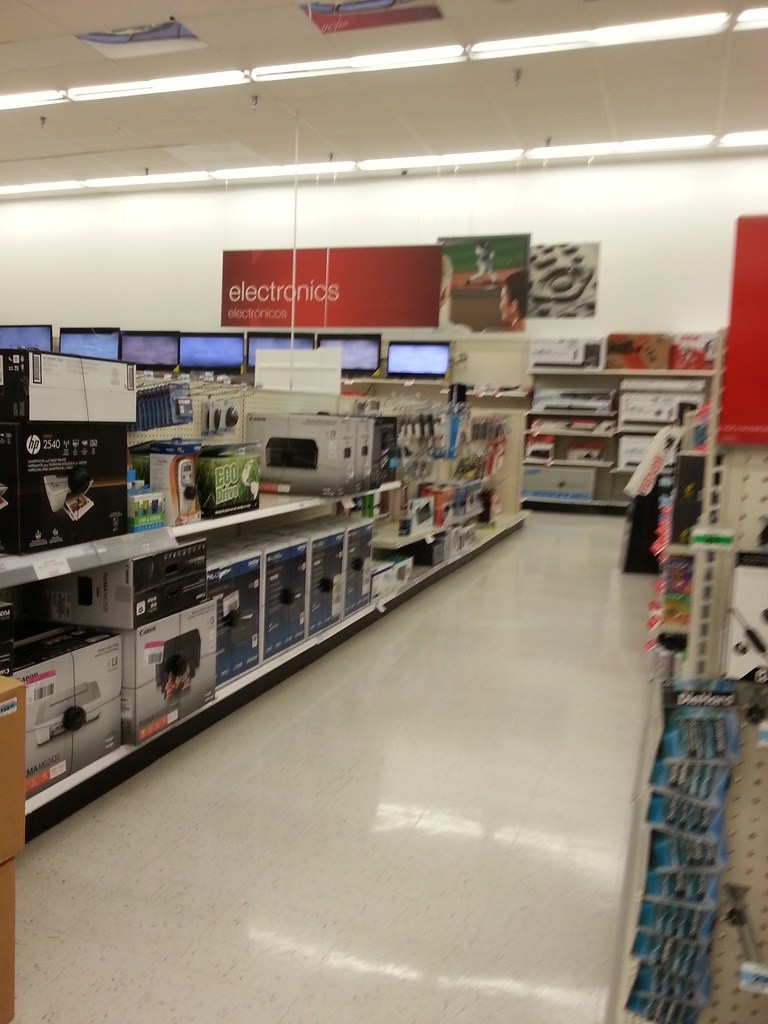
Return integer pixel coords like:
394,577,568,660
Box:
206,406,238,430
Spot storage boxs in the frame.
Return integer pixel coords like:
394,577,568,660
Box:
618,435,677,469
0,349,482,1024
671,333,716,369
526,435,554,463
566,444,608,463
617,379,706,435
607,335,669,368
530,337,607,369
530,418,616,436
624,425,685,498
531,389,617,413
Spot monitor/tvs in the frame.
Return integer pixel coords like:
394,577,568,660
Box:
386,341,451,379
58,327,381,376
0,325,53,353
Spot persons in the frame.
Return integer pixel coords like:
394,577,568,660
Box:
481,270,527,332
464,242,500,286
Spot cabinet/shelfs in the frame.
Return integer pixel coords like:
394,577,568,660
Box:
343,366,768,1024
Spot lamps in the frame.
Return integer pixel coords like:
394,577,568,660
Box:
0,8,768,201
